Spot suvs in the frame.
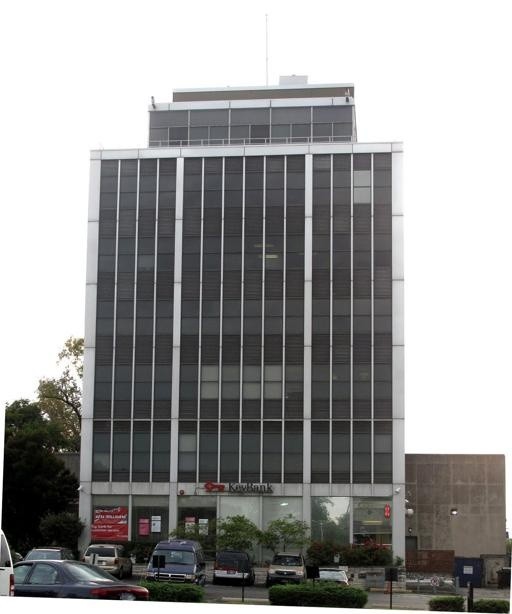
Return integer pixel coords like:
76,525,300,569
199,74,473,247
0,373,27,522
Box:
266,551,307,586
213,549,255,584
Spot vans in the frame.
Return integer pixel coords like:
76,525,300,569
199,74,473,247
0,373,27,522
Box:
146,538,206,587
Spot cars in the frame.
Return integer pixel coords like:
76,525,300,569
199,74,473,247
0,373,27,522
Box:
317,567,349,586
23,547,77,559
13,560,150,597
83,544,133,580
